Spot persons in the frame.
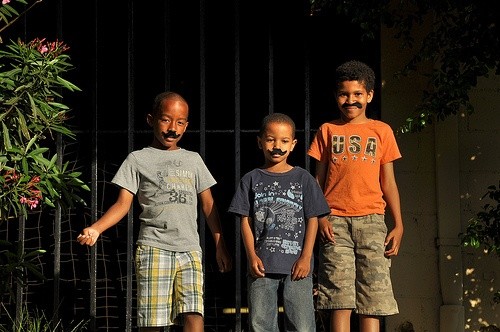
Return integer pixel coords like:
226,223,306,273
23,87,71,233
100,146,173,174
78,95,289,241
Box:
231,113,331,332
77,91,229,332
306,62,406,332
